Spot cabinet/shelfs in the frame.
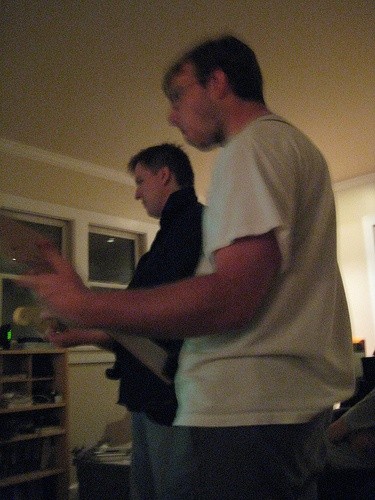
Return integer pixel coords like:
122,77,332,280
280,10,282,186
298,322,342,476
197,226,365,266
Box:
0,193,174,499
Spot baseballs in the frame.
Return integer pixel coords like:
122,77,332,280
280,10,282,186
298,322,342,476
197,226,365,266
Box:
14,306,31,324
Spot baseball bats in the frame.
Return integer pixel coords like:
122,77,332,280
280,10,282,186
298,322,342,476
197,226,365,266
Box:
0,216,179,385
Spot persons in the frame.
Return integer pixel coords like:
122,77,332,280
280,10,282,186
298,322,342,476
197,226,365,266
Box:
45,143,207,498
322,391,375,457
17,32,358,500
